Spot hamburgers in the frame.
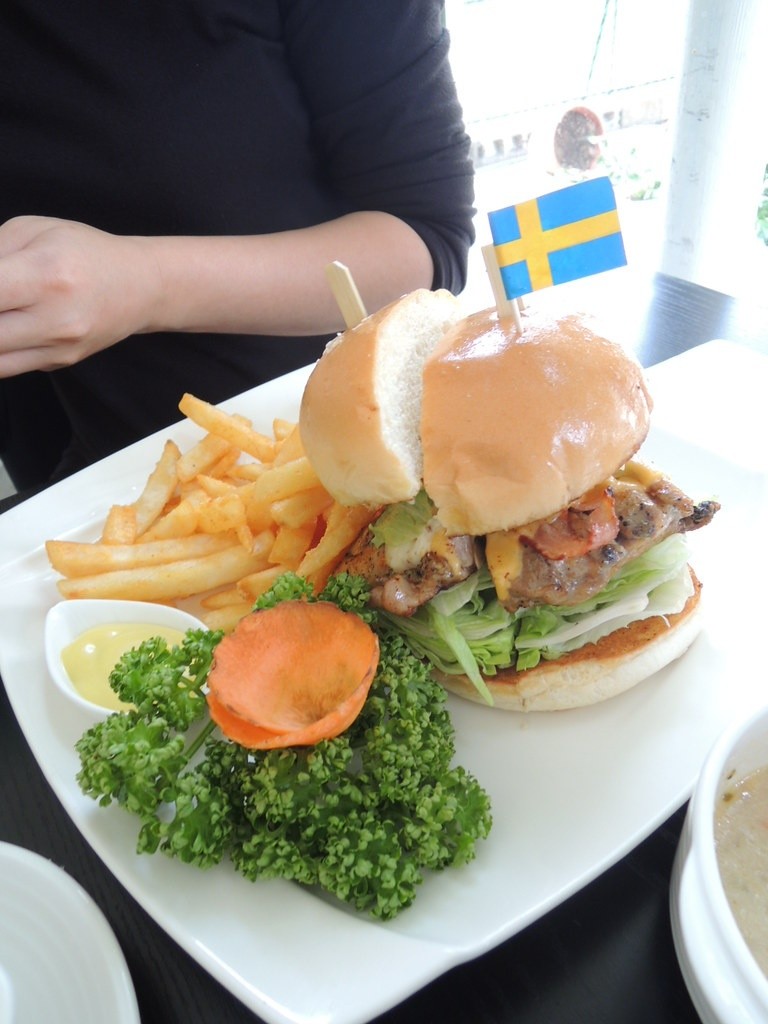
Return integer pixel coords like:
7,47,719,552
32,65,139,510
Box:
298,287,720,713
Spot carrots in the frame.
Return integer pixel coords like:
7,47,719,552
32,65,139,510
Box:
205,599,381,752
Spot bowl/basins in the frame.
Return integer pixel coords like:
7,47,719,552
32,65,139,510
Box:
669,706,767,1023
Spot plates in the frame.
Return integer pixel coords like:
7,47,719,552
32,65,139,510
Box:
0,840,141,1023
0,364,767,1024
45,599,209,714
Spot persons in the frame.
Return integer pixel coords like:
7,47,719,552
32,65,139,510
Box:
0,3,474,518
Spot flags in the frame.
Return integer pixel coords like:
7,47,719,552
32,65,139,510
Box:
489,175,630,300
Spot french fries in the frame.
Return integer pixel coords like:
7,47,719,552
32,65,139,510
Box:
42,392,378,635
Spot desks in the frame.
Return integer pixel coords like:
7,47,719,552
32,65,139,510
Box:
0,267,768,1024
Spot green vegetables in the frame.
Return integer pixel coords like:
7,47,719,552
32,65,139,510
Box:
72,571,494,920
